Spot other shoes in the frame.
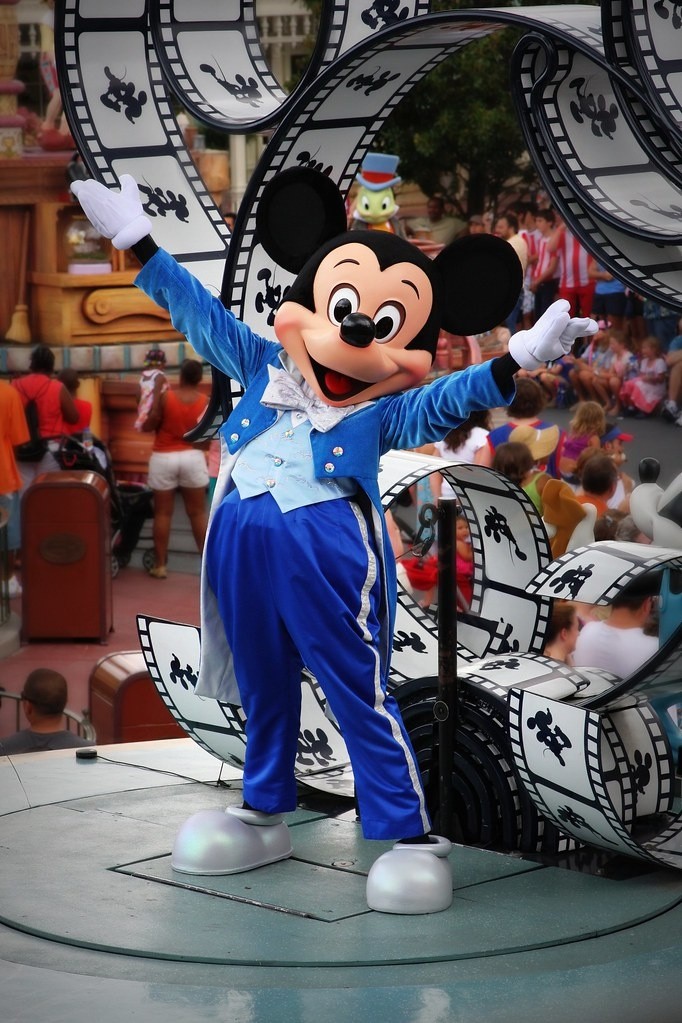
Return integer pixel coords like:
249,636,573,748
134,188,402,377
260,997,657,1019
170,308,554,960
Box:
659,400,682,428
149,565,169,579
2,575,22,599
569,402,581,412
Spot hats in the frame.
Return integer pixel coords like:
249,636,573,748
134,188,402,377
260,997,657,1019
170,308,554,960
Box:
599,425,634,445
509,423,560,461
143,350,165,365
469,213,485,226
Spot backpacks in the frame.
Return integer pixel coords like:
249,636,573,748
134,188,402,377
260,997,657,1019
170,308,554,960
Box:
12,378,57,462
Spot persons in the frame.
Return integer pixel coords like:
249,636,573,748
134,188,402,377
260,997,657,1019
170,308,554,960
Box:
0,668,95,757
574,577,678,726
0,347,94,597
516,320,682,425
415,376,652,610
545,602,579,665
135,349,214,577
402,196,682,349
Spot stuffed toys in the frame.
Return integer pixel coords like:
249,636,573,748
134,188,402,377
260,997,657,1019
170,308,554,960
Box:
351,153,406,237
71,167,600,913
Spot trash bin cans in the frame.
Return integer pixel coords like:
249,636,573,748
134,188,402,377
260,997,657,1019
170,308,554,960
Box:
88,649,192,745
21,471,115,645
